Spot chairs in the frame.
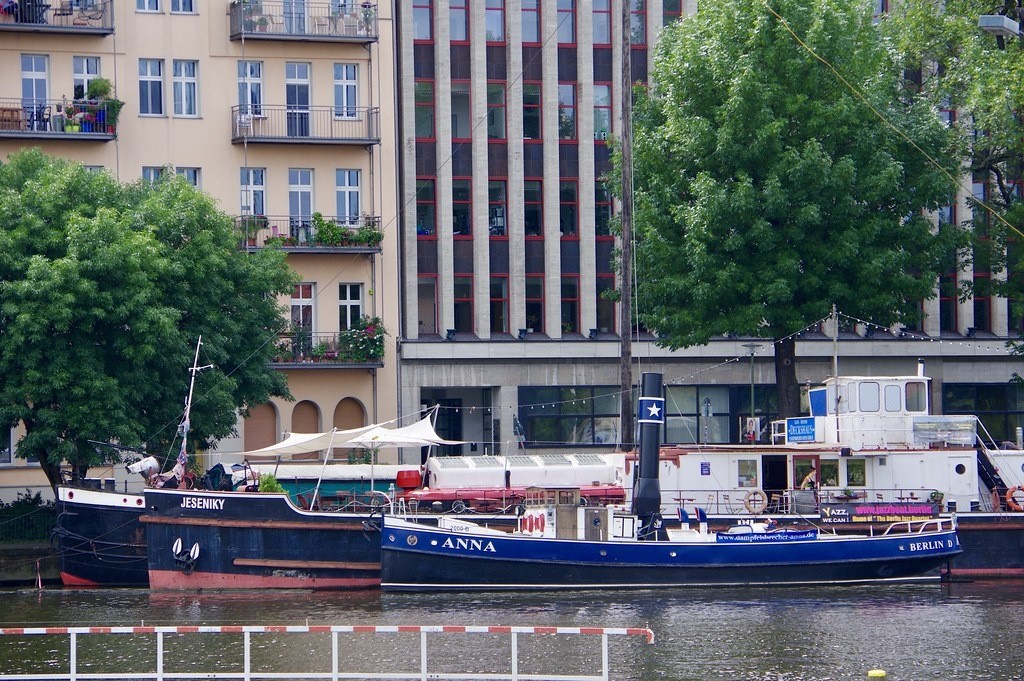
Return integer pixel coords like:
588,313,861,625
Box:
312,16,329,36
30,107,53,132
270,14,287,34
53,1,73,26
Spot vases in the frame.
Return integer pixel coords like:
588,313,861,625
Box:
278,354,373,363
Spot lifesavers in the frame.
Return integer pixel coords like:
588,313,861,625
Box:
1007,486,1024,511
534,512,545,536
743,489,769,514
520,510,533,535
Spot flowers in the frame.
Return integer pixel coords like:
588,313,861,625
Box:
272,334,296,351
339,314,387,359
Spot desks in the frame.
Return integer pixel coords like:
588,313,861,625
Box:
0,108,28,132
27,2,51,25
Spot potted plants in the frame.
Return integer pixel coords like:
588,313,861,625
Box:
243,16,268,33
302,211,385,246
64,77,124,134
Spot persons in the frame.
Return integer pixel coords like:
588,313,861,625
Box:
800,465,818,493
746,419,755,440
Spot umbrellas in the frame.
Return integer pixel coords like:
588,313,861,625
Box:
334,425,440,492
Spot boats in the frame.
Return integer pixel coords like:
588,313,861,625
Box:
54,302,1024,590
